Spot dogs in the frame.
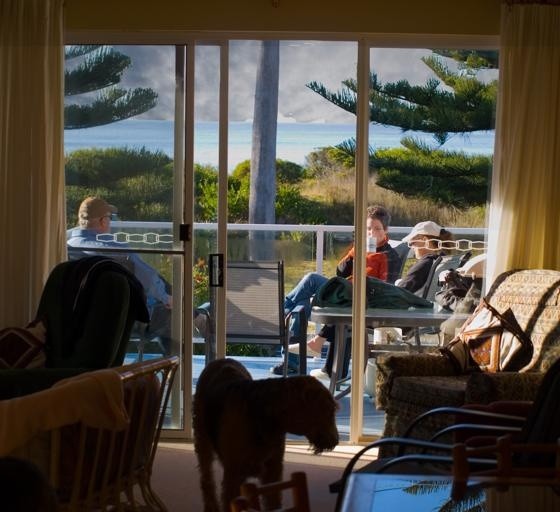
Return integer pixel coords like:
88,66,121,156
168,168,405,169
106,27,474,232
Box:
192,359,340,512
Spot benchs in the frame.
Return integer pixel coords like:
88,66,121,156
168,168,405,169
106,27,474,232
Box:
0,355,181,512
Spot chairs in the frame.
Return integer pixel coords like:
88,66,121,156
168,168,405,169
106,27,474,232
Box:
374,270,560,469
385,240,411,283
35,257,144,369
329,358,559,512
335,252,472,400
194,260,307,378
70,251,170,362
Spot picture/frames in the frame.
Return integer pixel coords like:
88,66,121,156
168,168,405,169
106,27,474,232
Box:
464,328,502,373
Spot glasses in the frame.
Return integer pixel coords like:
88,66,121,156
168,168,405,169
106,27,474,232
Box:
100,214,113,222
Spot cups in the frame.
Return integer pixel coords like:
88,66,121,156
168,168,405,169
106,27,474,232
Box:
368,235,378,254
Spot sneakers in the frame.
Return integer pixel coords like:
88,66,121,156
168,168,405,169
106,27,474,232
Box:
270,363,298,376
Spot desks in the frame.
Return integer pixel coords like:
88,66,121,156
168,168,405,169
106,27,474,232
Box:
313,310,451,396
333,473,560,511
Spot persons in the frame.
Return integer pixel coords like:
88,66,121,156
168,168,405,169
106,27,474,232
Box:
65,192,207,359
270,204,404,377
286,218,458,383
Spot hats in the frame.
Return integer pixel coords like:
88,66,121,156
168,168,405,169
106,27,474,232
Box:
401,220,444,243
75,196,118,220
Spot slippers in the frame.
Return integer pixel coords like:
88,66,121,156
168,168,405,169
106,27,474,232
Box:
285,341,323,359
309,368,332,384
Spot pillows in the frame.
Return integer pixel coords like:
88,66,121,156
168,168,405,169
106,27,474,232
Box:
0,328,46,370
